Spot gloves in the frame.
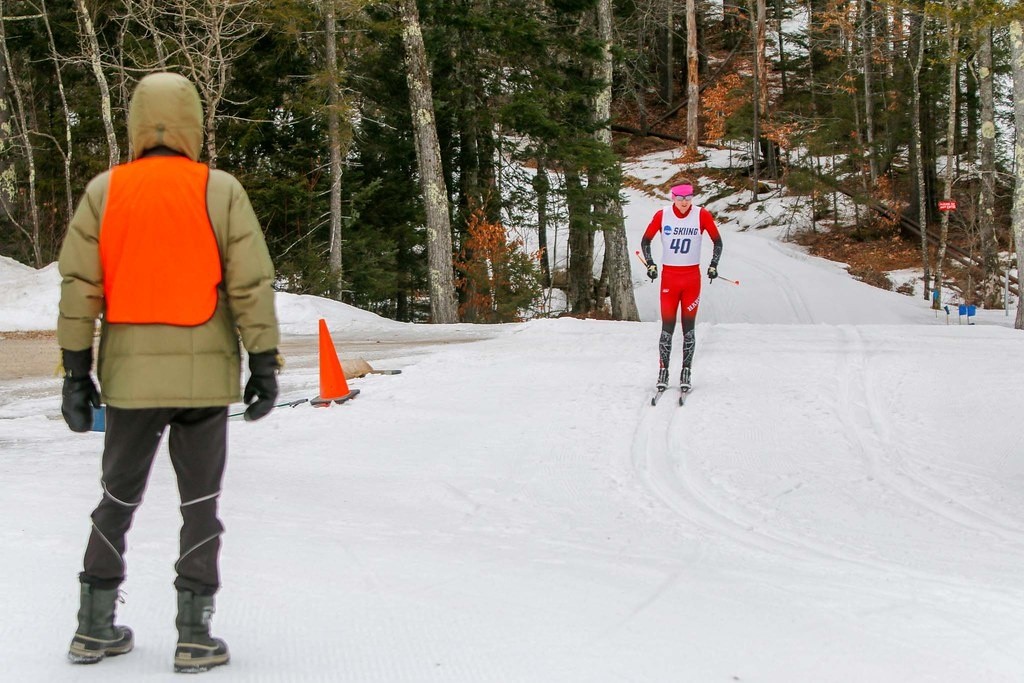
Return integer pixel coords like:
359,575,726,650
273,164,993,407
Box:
707,263,718,279
243,348,284,421
52,346,101,432
647,264,657,279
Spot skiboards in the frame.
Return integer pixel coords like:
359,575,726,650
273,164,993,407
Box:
651,383,694,406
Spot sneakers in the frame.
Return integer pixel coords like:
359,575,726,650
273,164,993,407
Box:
680,367,691,392
656,367,669,391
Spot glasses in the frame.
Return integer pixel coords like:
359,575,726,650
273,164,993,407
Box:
671,191,693,201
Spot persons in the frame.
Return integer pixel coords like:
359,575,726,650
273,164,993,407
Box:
641,177,723,390
59,72,287,674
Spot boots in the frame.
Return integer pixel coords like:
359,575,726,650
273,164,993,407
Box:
68,583,134,664
174,590,230,674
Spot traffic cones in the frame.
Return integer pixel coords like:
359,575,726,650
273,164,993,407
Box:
309,317,361,407
